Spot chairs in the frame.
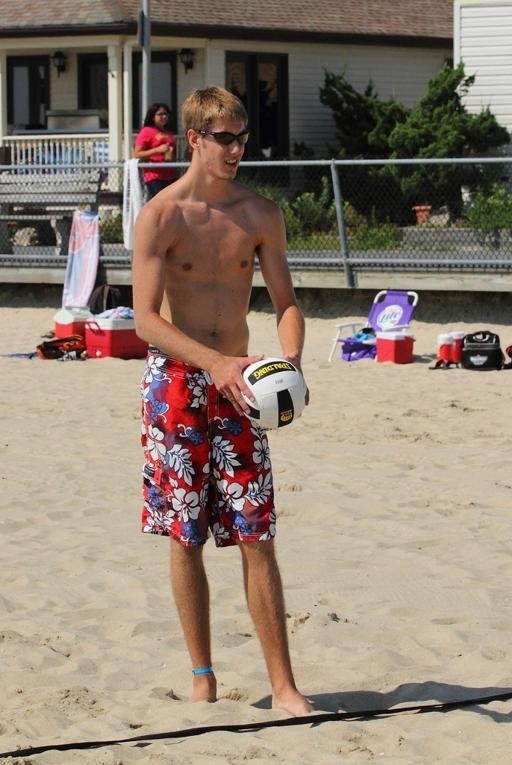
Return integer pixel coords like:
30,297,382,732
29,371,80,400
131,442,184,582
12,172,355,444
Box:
323,288,418,365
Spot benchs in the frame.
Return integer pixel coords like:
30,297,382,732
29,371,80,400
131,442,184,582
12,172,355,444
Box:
1,170,101,258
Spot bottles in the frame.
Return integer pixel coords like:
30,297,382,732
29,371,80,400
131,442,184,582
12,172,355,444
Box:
438,332,464,364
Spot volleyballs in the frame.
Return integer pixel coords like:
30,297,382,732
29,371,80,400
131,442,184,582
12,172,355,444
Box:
241,358,307,429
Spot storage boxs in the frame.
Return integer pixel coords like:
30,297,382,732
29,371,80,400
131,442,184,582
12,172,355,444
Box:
374,332,413,364
56,305,147,362
438,332,468,363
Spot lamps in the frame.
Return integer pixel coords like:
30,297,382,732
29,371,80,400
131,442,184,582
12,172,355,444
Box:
178,47,194,74
50,51,66,77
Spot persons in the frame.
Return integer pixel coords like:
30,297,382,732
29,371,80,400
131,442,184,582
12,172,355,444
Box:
133,102,178,198
132,86,317,718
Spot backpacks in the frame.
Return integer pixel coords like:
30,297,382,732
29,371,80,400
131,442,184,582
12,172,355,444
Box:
91,285,121,314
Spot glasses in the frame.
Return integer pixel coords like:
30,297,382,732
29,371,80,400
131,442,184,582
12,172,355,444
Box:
200,129,251,148
155,111,170,117
